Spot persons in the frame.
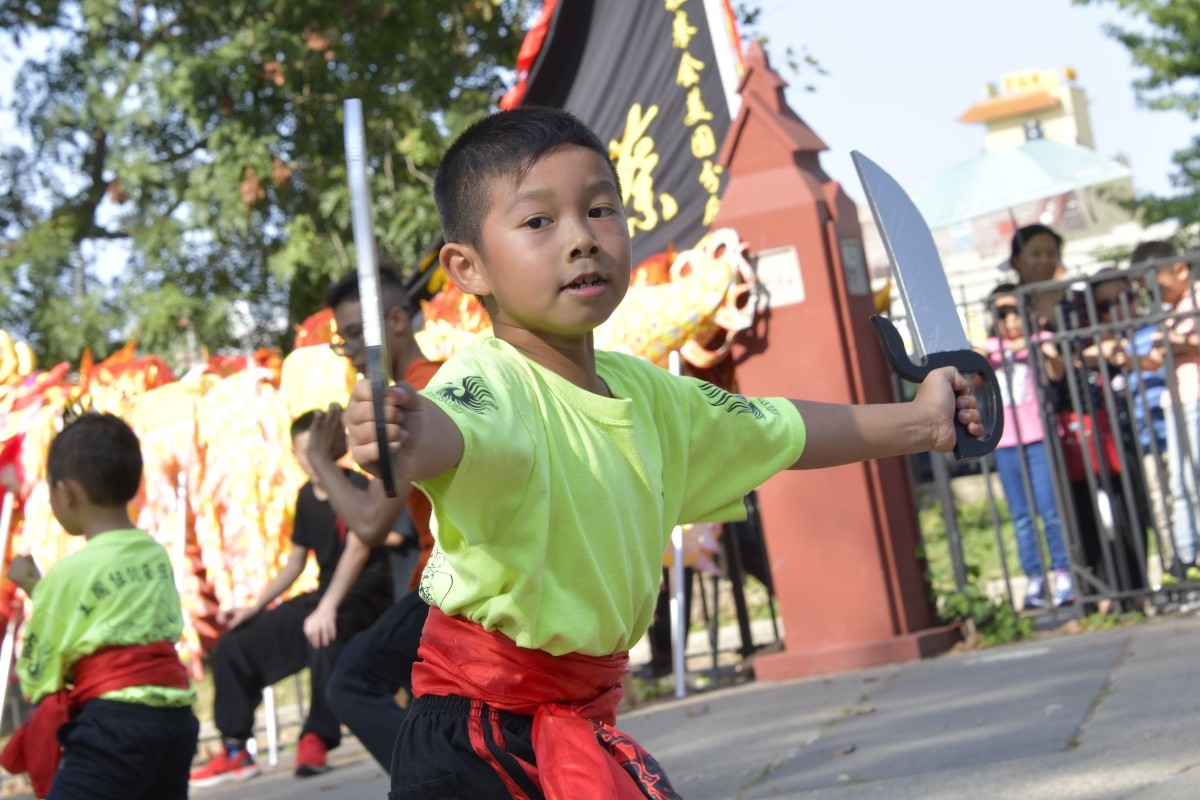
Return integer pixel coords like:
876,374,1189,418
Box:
353,108,985,800
186,411,398,786
306,259,449,603
975,222,1200,617
7,412,204,800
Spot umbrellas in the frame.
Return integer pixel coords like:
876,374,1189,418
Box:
916,140,1131,231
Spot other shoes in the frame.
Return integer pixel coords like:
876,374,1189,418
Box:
1160,558,1200,588
1024,575,1047,610
1053,566,1075,607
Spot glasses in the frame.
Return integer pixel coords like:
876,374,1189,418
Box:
1096,293,1135,313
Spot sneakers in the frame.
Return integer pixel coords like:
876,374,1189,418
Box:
294,732,328,777
188,748,262,789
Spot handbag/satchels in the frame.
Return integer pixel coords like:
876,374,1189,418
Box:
1055,409,1123,481
